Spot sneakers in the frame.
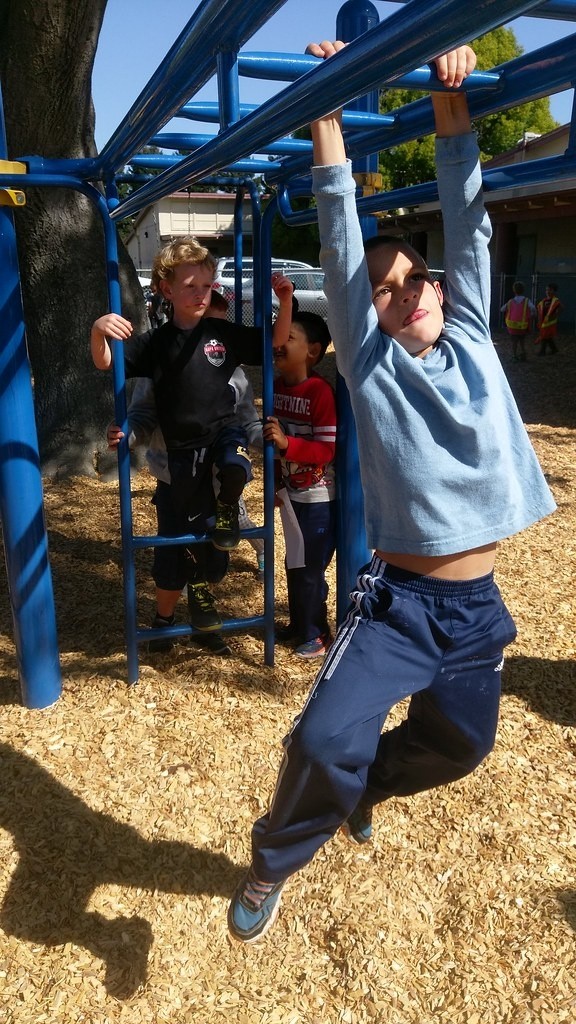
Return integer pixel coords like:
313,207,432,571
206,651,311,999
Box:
186,581,224,632
345,804,374,845
187,627,232,655
226,862,292,944
145,613,178,659
292,629,334,658
210,494,241,551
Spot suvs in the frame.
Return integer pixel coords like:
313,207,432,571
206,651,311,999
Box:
213,257,318,294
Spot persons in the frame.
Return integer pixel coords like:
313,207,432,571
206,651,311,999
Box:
93,235,294,658
262,310,338,658
228,39,558,943
534,283,564,356
500,282,538,362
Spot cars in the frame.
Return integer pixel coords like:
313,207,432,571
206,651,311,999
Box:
229,272,329,325
137,269,232,316
